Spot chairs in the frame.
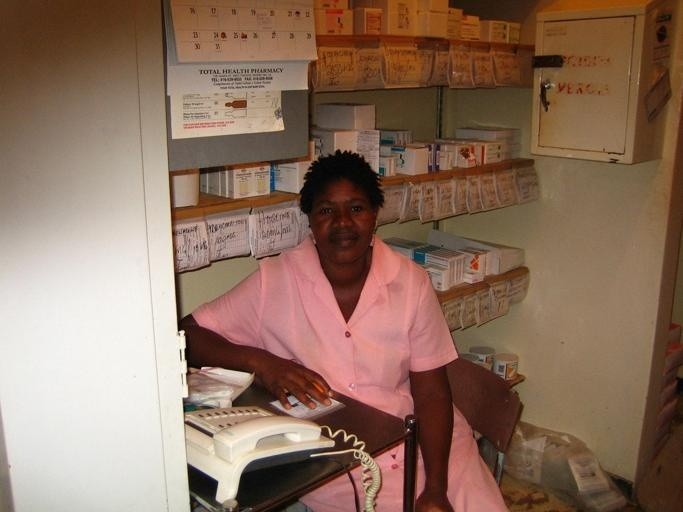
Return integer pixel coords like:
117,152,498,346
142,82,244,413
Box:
444,360,525,490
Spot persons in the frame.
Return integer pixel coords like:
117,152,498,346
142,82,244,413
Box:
179,148,509,512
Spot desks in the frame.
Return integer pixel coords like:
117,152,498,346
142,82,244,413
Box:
187,383,414,510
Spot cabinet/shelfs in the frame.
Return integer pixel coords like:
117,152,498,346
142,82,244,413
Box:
163,27,536,305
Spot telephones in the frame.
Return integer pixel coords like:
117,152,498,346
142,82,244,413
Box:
184,406,334,504
